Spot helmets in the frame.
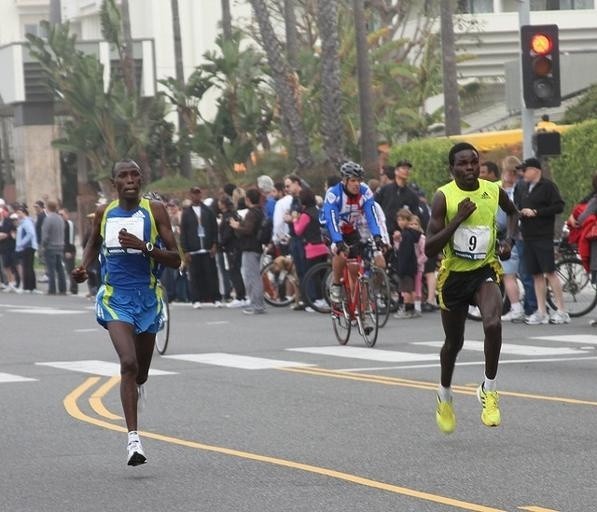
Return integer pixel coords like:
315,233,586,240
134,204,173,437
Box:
341,163,365,179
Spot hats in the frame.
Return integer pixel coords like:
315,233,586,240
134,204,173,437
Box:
516,159,541,169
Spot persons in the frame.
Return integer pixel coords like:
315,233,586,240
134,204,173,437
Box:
0,194,78,294
154,174,341,314
423,142,519,434
569,175,597,325
481,158,572,326
71,158,181,465
368,159,438,321
319,161,391,325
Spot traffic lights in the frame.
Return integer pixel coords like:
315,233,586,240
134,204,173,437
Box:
520,23,560,109
535,130,562,159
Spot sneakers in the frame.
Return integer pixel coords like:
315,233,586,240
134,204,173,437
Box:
137,385,147,408
525,309,548,324
329,282,342,304
394,301,437,319
550,311,570,325
126,436,146,467
306,300,329,312
503,309,525,321
226,300,262,315
436,394,457,433
478,383,501,428
358,320,372,336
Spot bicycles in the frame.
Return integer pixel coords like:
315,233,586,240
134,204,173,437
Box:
256,236,399,346
144,259,170,354
464,239,596,323
